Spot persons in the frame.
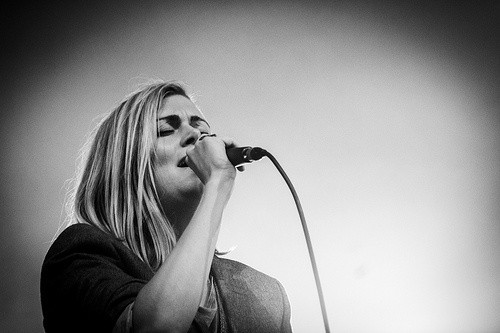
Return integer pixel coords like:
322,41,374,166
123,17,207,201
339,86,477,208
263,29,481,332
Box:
39,81,295,333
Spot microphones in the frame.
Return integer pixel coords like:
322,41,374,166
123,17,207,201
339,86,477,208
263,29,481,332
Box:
185,146,267,168
